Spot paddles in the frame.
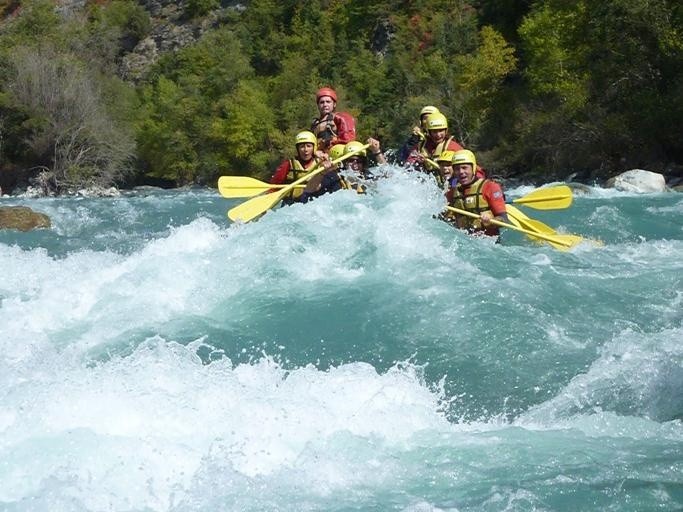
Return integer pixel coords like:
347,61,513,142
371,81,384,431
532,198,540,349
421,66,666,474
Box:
218,176,307,198
228,143,370,223
503,185,573,210
445,204,602,250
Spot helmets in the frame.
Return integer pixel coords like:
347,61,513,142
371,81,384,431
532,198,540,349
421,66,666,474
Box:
342,140,366,158
425,113,447,131
418,106,439,119
433,151,455,163
294,131,317,156
450,149,476,175
316,88,336,105
327,144,345,169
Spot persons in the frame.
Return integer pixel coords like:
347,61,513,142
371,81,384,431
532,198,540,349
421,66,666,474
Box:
265,87,485,207
438,150,508,242
